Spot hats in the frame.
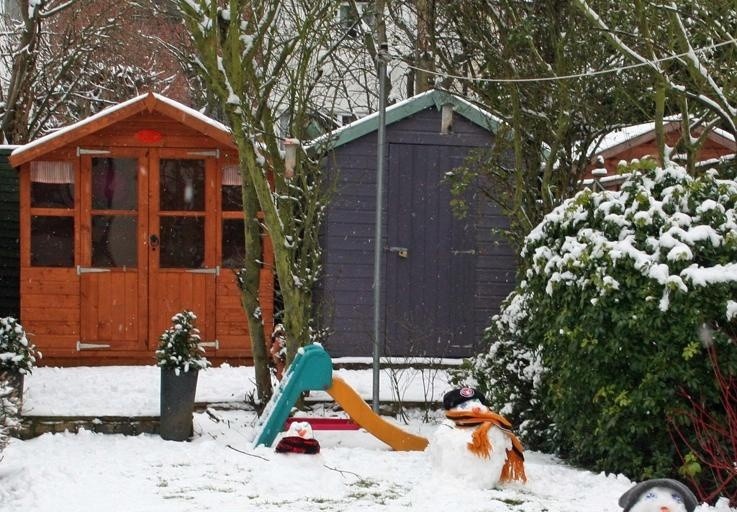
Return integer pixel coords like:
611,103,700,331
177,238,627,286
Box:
440,387,486,410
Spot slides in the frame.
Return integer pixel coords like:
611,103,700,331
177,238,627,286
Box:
326,376,428,451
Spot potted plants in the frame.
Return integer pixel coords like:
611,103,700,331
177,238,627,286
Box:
0,316,42,440
153,309,213,442
